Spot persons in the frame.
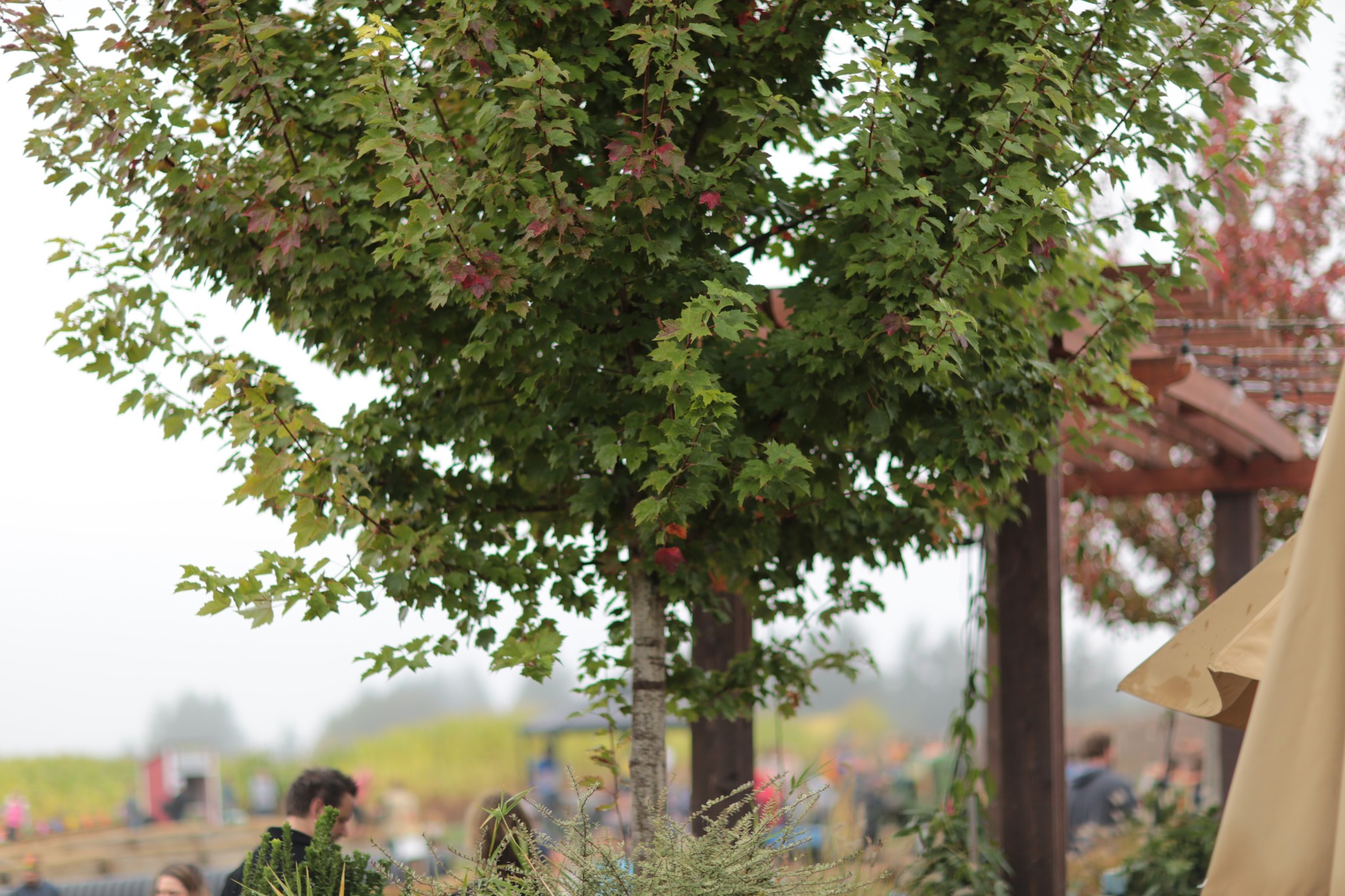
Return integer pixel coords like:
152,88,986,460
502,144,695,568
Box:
448,791,548,896
152,865,214,896
1060,727,1140,853
8,867,61,896
1142,747,1201,832
524,729,991,875
220,766,482,896
